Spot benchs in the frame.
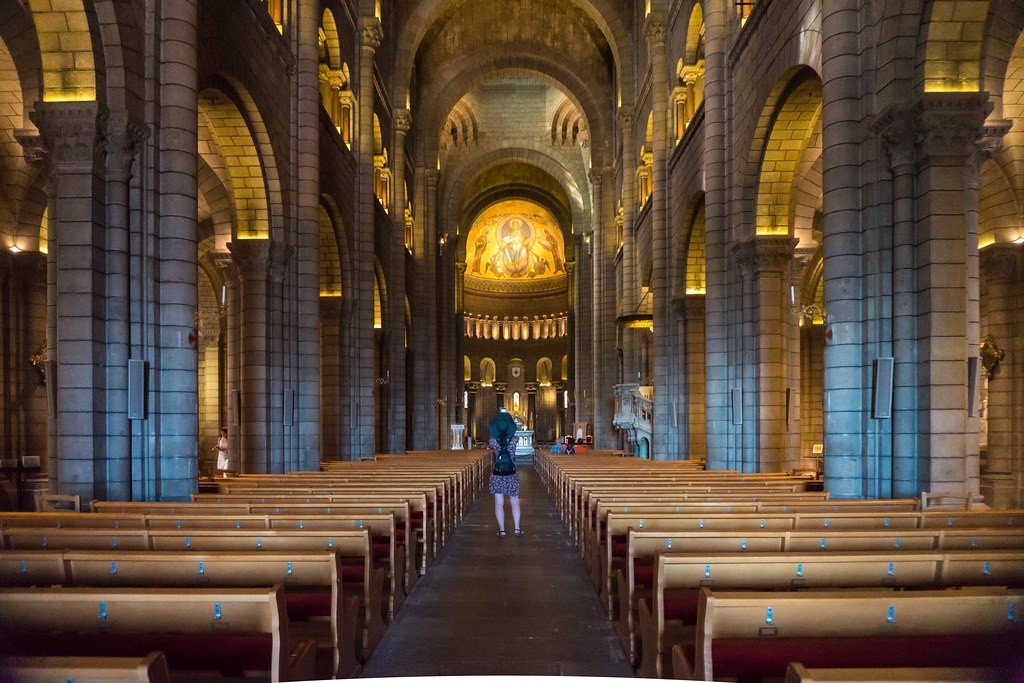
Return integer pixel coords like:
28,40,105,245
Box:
2,446,1024,683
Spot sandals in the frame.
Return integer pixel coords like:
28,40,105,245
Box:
496,529,506,536
514,528,525,536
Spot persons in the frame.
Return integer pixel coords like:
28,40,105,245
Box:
213,426,229,478
488,412,525,536
550,438,588,454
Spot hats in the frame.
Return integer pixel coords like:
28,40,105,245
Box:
488,413,518,439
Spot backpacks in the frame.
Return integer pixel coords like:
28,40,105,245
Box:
493,438,517,475
